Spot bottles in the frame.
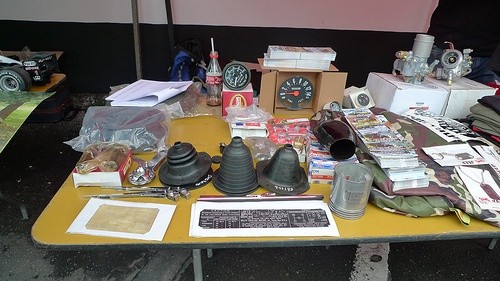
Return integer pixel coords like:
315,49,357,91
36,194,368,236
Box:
205,51,223,106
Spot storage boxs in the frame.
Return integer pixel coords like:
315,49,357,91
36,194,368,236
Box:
365,71,497,126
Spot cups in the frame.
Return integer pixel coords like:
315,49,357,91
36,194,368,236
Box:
328,162,375,221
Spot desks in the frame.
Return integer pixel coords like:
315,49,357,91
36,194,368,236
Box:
0,72,67,205
30,91,499,281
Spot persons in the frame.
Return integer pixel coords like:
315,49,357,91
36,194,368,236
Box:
427,0,500,84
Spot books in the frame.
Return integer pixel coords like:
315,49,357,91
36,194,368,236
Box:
72,151,134,187
229,121,270,138
